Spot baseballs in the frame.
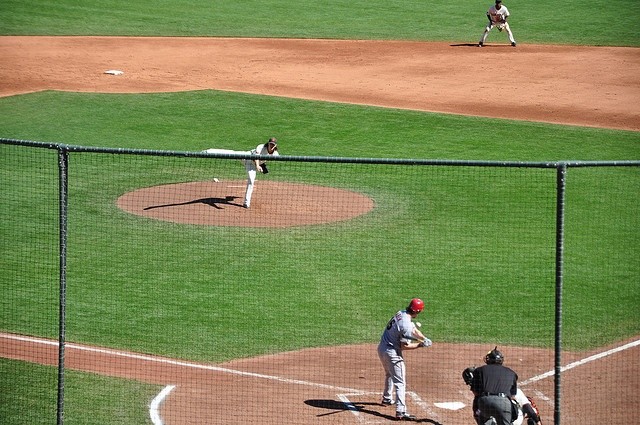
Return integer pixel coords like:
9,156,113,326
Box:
416,322,421,327
212,177,219,183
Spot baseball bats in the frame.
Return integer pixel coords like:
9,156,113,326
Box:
401,333,426,343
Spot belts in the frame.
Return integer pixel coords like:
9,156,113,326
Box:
474,393,508,399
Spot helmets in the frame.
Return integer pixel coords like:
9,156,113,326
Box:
483,346,504,365
462,366,475,386
408,298,425,312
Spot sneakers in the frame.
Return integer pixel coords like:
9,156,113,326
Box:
476,42,483,47
201,149,207,153
381,400,396,406
396,412,415,419
243,204,250,208
511,42,516,46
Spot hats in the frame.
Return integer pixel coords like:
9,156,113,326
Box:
268,137,277,146
495,0,501,3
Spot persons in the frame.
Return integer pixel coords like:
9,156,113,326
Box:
462,368,539,425
470,350,518,425
200,137,281,208
378,298,432,420
479,0,516,47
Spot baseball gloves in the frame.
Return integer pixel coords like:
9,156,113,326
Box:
496,23,506,31
